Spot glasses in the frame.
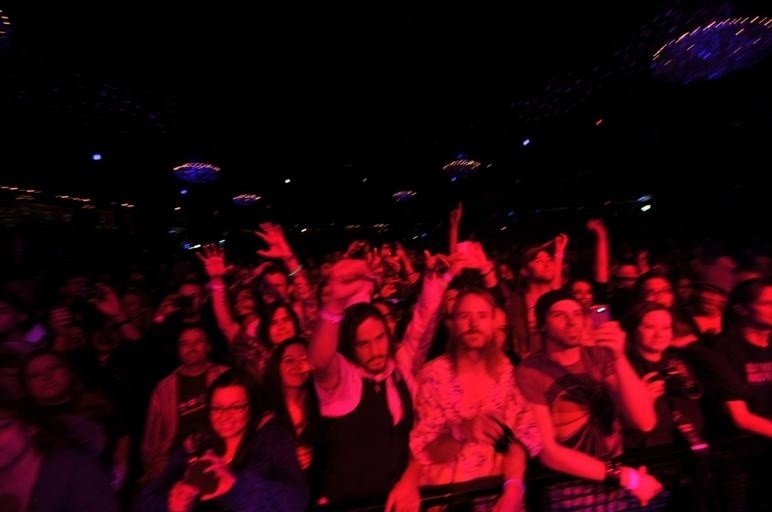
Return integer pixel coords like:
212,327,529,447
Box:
208,401,253,414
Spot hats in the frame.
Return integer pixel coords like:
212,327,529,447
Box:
535,288,585,329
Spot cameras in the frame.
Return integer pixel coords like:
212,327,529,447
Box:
590,304,610,324
195,434,226,457
